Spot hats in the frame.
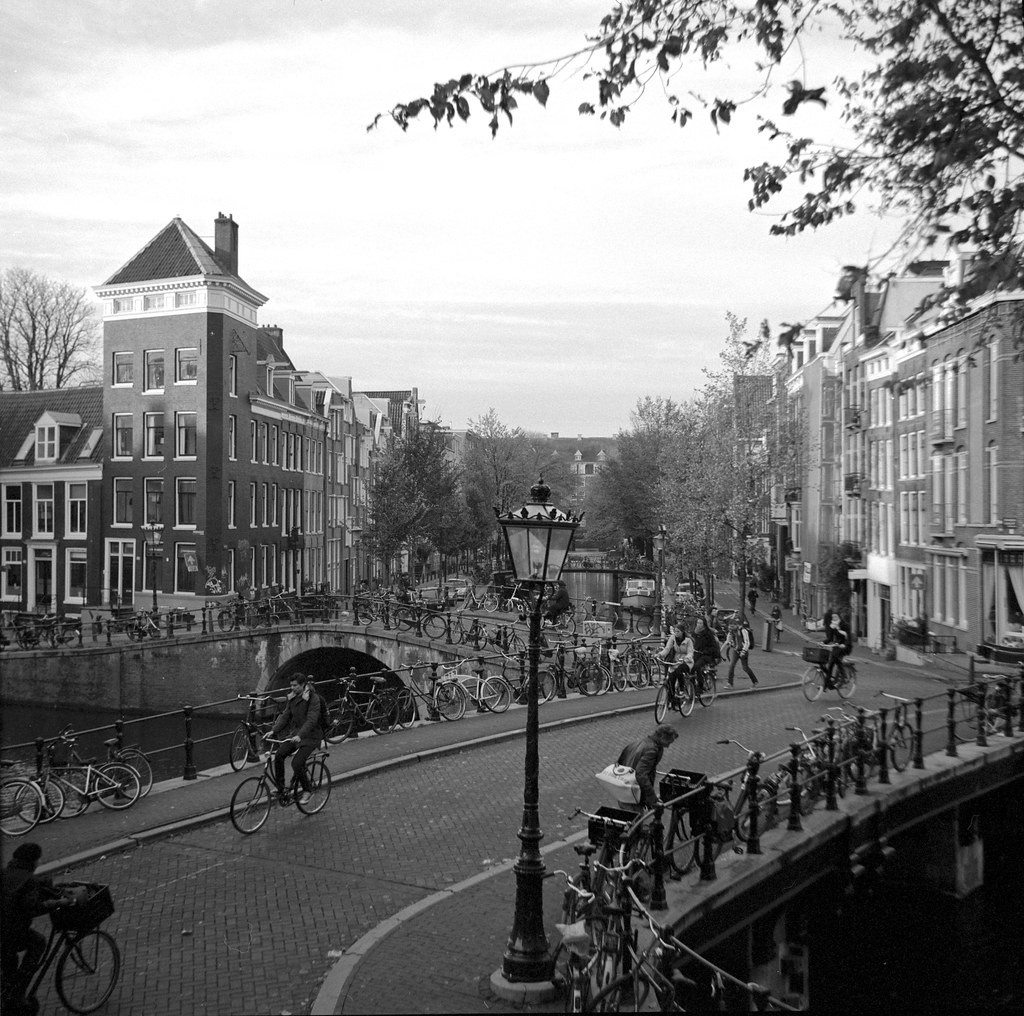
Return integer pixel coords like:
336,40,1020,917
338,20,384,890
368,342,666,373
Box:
14,843,41,859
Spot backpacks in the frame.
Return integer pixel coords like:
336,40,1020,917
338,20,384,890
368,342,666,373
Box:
307,696,330,732
740,627,754,649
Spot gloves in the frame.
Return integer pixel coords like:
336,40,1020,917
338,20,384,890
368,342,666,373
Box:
57,897,71,908
740,650,746,658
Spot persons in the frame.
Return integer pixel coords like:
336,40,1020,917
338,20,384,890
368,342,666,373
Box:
691,616,719,693
747,586,758,616
618,724,679,861
820,612,852,692
655,624,694,709
721,619,759,690
771,605,782,620
263,673,324,805
0,842,73,1016
396,572,415,605
543,579,570,625
823,609,833,638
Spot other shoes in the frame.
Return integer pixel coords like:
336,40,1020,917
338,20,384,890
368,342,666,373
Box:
833,683,840,689
271,789,279,796
299,791,315,804
544,619,552,625
750,681,759,689
724,683,734,690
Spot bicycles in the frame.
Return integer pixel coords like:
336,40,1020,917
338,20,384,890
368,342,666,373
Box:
11,724,143,826
231,693,288,774
0,576,916,1016
0,758,50,836
230,736,331,833
803,639,859,703
942,661,1024,744
43,724,156,819
0,882,123,1015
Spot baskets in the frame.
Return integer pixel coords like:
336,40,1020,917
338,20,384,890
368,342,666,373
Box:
660,768,707,806
589,806,642,845
52,882,114,930
802,647,830,663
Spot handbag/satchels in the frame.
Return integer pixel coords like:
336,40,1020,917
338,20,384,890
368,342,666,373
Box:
664,647,675,677
593,762,641,803
776,621,783,631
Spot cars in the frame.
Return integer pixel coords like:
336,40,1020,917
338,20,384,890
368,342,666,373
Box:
419,587,451,613
711,609,752,643
434,584,458,607
447,579,471,602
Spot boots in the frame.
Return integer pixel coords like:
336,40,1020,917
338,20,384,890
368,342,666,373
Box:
5,971,34,1011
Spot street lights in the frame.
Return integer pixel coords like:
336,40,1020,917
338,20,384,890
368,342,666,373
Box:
350,525,365,627
651,524,671,641
491,474,583,1007
140,516,168,638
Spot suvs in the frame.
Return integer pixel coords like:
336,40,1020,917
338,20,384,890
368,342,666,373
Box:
675,581,705,602
